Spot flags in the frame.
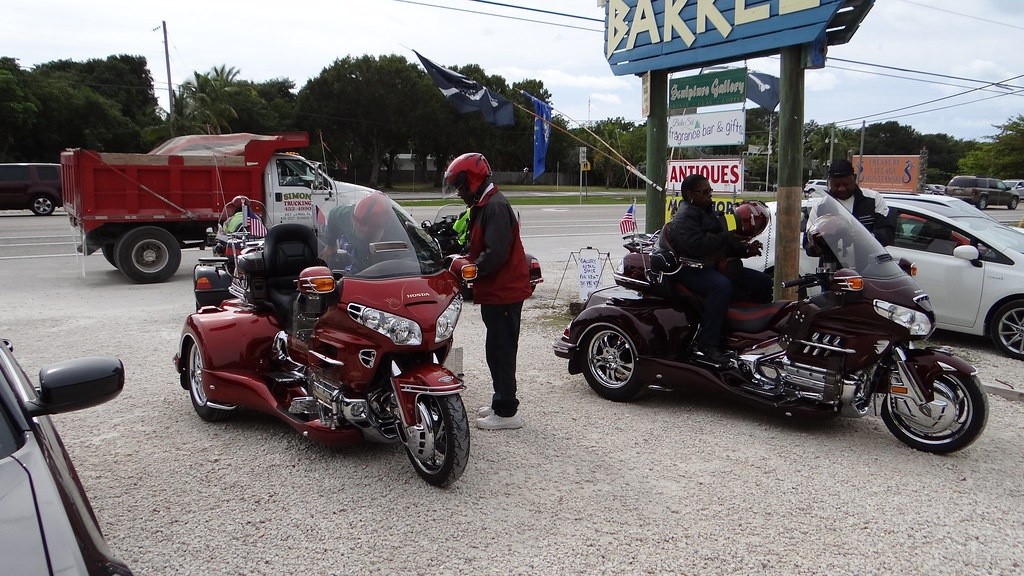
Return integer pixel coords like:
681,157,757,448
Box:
620,204,637,235
700,66,781,113
415,51,514,126
247,206,267,237
522,92,551,180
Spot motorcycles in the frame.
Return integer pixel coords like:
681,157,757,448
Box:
553,189,991,454
419,203,545,300
171,191,479,488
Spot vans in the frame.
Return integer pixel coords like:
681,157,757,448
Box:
0,162,63,216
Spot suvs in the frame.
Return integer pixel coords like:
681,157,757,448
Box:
803,179,828,194
943,175,1020,211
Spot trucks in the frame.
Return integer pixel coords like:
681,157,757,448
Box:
59,131,390,284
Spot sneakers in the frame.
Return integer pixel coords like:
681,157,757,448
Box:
479,403,495,417
476,406,522,430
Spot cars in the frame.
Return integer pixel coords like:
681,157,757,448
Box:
1002,179,1024,204
740,192,1024,361
0,332,134,575
924,184,947,195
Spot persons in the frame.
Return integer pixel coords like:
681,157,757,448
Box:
802,159,897,415
223,203,247,233
446,206,471,268
669,174,771,363
442,153,533,430
326,194,392,429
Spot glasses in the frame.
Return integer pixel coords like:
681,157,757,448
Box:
696,189,714,194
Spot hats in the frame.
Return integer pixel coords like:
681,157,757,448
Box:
806,212,850,257
730,199,771,237
828,161,854,179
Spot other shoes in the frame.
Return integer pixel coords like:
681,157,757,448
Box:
694,346,731,365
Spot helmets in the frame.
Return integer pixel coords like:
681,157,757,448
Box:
440,153,493,195
352,195,392,235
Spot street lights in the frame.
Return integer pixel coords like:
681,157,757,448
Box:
825,128,838,164
